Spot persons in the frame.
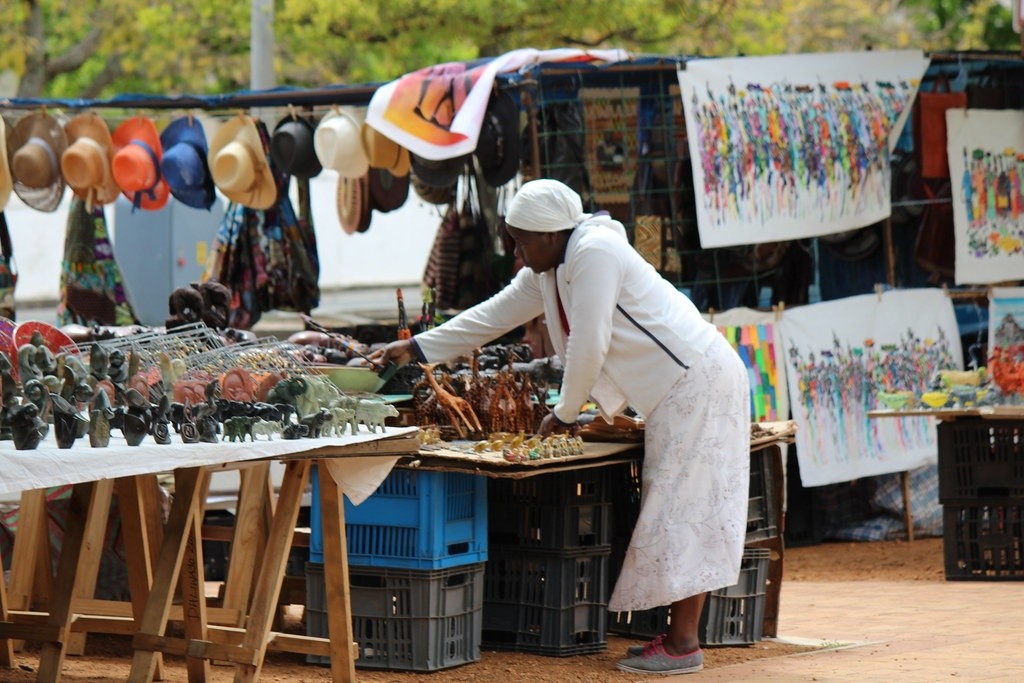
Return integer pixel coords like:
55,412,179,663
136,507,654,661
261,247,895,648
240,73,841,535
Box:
361,179,751,676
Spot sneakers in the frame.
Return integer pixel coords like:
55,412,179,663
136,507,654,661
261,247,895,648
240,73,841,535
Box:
627,633,667,658
616,643,704,675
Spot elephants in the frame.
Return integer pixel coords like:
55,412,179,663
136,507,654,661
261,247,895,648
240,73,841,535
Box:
266,373,341,416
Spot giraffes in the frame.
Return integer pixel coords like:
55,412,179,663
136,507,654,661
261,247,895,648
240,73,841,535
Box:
411,346,550,439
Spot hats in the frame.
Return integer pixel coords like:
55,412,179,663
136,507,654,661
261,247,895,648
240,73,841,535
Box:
361,118,411,177
270,113,322,179
313,107,369,178
59,115,121,215
337,157,465,233
110,116,170,216
470,87,520,186
208,115,277,209
7,114,69,213
157,115,217,210
0,113,13,213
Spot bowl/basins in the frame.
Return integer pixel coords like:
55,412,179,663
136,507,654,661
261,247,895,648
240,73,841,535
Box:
307,366,387,396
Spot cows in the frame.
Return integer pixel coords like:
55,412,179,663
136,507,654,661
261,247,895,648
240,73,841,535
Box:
321,396,402,437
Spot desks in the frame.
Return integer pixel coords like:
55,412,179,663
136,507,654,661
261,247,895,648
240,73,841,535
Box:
0,428,418,682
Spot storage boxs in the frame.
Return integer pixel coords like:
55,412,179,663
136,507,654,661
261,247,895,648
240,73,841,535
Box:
306,443,782,675
937,418,1024,584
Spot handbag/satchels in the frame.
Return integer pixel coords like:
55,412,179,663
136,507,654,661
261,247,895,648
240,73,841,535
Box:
425,178,527,341
